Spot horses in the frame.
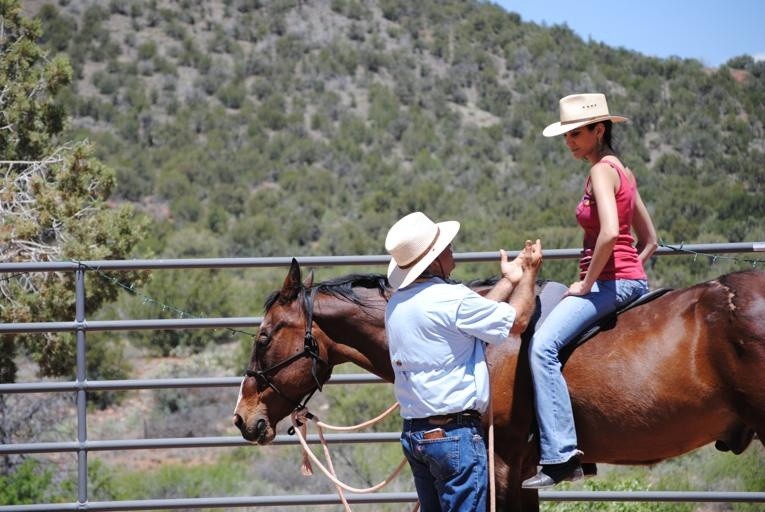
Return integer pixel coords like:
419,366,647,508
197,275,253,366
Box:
233,257,765,512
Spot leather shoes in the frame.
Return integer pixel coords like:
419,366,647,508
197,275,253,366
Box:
522,455,596,489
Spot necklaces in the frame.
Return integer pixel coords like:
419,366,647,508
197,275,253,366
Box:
590,152,618,166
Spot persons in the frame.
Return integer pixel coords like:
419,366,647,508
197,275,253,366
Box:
521,93,659,489
385,212,543,512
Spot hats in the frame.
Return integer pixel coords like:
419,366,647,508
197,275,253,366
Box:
385,212,460,289
543,94,629,138
